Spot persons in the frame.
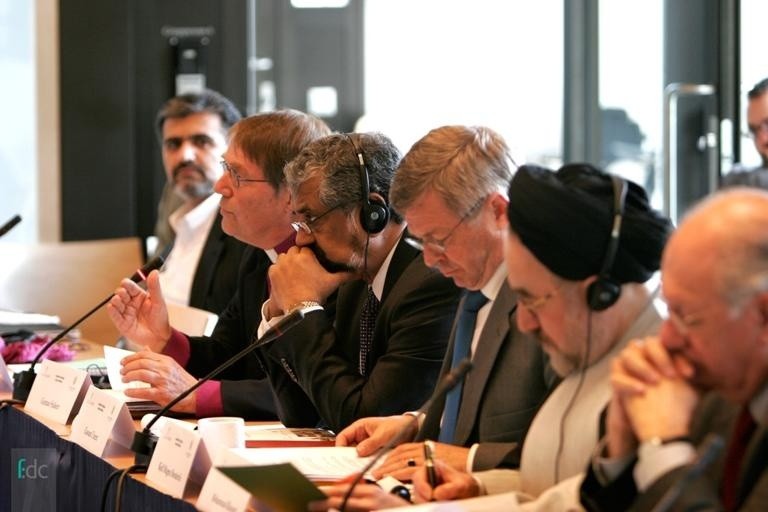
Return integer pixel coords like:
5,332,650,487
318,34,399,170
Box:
127,87,243,340
252,132,459,437
725,78,767,186
335,126,565,485
308,163,665,512
106,107,335,422
575,185,766,512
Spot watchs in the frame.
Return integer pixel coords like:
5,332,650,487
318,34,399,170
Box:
630,435,696,453
282,300,320,315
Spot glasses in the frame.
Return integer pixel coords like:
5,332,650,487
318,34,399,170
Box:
742,115,768,141
402,194,487,252
219,159,276,188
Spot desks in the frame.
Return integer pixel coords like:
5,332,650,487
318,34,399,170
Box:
0,329,281,512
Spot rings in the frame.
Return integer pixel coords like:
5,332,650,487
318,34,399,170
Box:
405,457,416,467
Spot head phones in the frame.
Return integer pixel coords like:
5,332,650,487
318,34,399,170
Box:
588,174,628,310
346,132,386,234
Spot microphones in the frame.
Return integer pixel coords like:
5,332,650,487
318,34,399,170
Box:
129,308,305,470
12,256,164,401
0,216,21,236
339,359,473,512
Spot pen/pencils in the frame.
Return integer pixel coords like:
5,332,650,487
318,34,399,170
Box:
423,441,437,488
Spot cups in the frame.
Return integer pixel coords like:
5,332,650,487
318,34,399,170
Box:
196,415,246,469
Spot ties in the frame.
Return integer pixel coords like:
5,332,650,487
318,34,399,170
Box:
718,402,762,512
436,288,493,446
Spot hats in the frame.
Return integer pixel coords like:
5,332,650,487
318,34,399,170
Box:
507,163,678,286
